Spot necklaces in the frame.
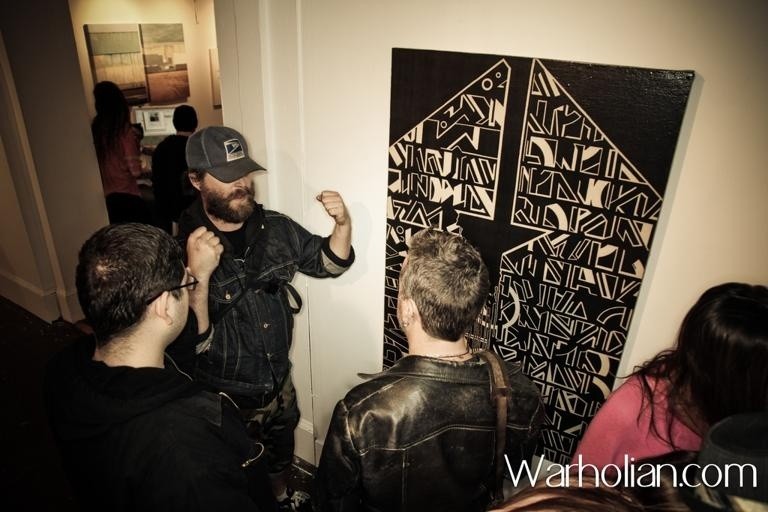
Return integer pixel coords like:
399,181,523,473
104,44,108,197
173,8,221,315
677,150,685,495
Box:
433,348,471,361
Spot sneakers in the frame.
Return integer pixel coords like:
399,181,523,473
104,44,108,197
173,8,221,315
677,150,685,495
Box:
277,486,311,511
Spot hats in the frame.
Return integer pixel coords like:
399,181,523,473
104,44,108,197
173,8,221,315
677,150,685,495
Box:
185,127,266,183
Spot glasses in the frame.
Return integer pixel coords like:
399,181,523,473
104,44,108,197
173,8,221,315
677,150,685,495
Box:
146,275,199,305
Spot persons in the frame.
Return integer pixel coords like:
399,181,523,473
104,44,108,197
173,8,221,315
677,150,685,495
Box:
92,80,149,223
176,125,357,512
153,105,199,213
1,226,276,512
314,229,542,510
566,282,767,481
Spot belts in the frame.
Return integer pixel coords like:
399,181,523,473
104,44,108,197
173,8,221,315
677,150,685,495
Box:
244,376,291,410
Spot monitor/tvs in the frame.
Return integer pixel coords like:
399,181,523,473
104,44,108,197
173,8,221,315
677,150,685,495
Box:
129,105,185,145
